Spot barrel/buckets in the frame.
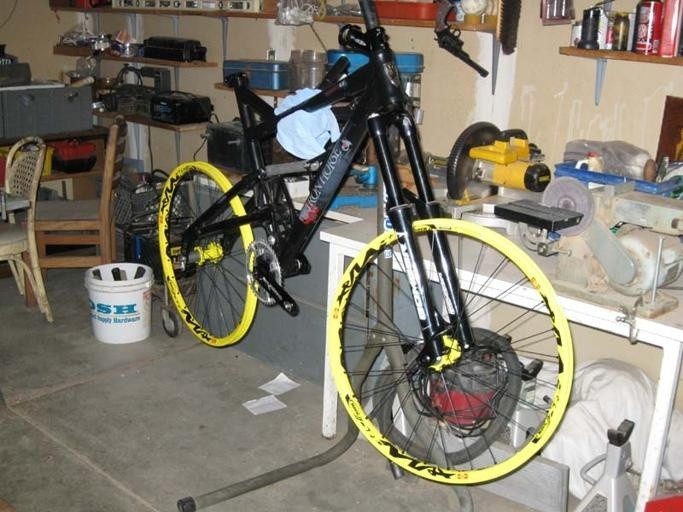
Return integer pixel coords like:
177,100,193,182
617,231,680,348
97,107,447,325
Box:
84,263,154,345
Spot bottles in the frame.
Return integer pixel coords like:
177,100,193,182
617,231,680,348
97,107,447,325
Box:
613,12,629,51
91,266,145,280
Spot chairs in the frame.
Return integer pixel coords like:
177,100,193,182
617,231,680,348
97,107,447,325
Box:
21,123,119,308
0,136,55,323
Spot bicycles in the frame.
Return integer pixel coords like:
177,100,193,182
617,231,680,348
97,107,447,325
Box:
158,0,575,486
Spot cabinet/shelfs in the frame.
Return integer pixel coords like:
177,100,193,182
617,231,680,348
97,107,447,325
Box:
557,0,680,107
50,5,228,179
0,126,109,280
213,8,502,112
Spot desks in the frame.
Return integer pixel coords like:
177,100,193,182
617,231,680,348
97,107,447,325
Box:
320,219,683,512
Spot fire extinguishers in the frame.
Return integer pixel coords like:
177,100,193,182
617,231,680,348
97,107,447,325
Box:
632,0,663,55
